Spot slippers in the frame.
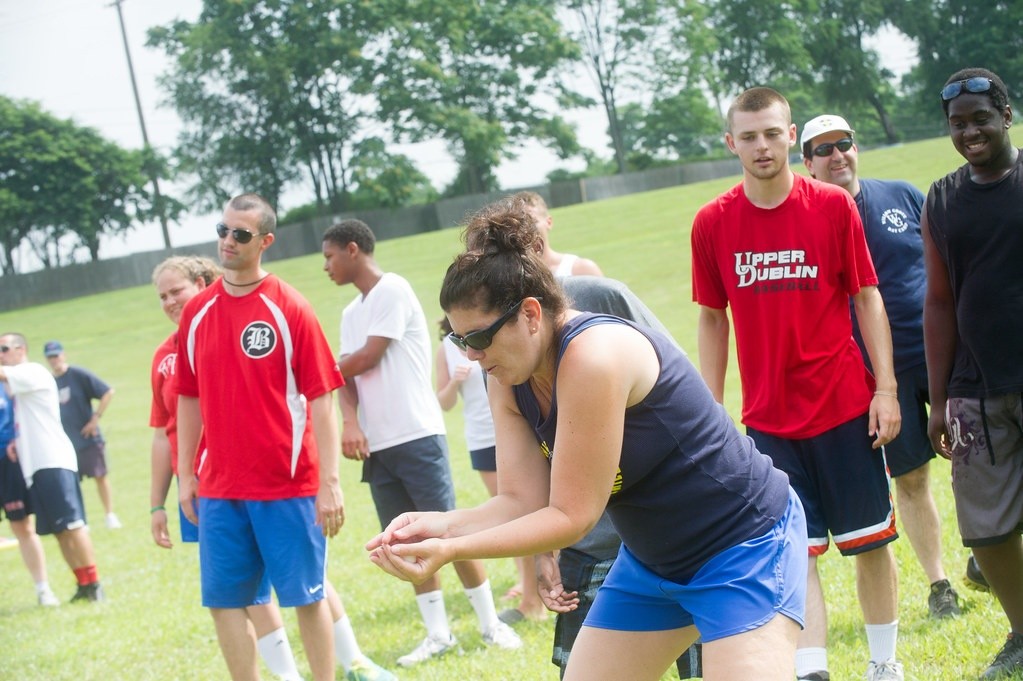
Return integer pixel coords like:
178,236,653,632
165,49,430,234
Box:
500,609,550,626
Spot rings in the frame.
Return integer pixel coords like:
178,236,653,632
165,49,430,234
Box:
336,516,341,520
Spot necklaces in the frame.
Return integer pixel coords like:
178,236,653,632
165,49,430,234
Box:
222,275,269,287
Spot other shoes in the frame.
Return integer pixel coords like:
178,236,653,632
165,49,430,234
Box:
62,583,98,605
500,582,523,604
37,583,60,608
343,659,401,681
106,513,121,529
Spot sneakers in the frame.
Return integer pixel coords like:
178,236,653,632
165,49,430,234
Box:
863,657,904,681
797,670,830,681
978,629,1023,681
397,633,457,668
963,554,996,596
928,579,961,623
478,619,523,653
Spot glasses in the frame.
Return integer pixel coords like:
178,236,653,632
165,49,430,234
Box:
448,296,543,351
0,347,9,353
810,138,852,156
940,77,996,100
216,224,267,244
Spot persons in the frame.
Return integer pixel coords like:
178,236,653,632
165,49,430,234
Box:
690,88,907,681
0,333,123,606
323,220,524,668
367,191,808,681
920,68,1023,681
800,113,993,620
148,194,346,681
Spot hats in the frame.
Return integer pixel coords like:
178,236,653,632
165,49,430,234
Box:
45,341,63,357
800,114,855,160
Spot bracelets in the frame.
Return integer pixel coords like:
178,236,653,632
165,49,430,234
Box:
93,412,102,417
873,391,897,398
150,505,165,514
